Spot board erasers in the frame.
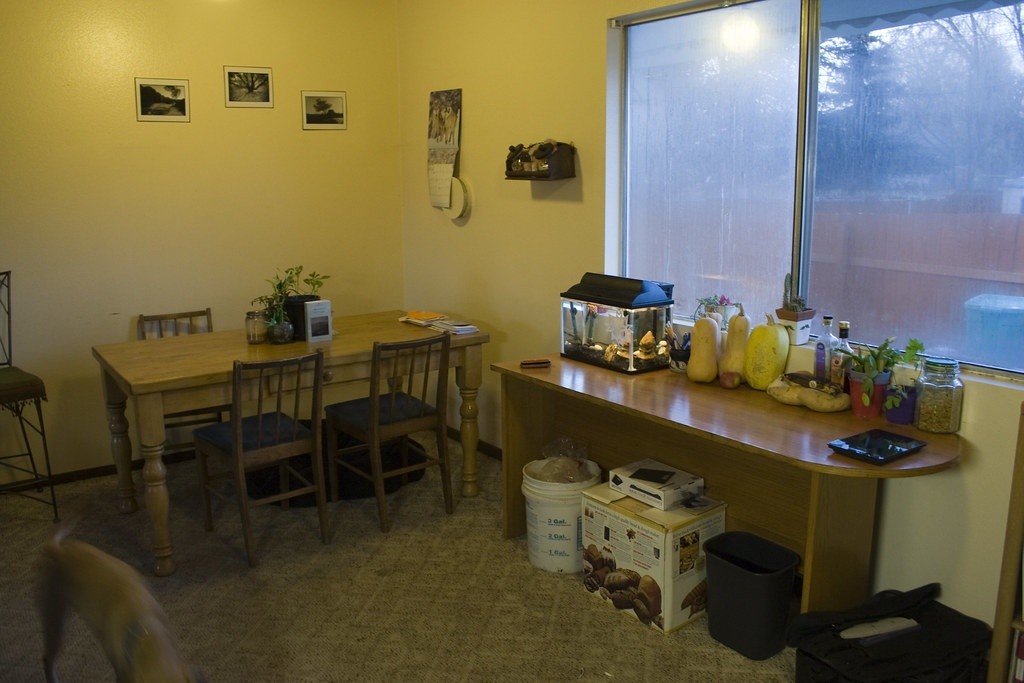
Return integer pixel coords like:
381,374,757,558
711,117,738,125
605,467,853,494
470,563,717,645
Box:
520,358,551,369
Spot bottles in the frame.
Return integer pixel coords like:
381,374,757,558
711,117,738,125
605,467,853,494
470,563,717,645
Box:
913,358,963,434
245,311,267,344
813,316,839,385
506,139,557,172
829,320,854,392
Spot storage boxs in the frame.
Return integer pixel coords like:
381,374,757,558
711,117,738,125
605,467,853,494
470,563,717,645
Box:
579,481,729,636
609,458,704,511
559,297,674,376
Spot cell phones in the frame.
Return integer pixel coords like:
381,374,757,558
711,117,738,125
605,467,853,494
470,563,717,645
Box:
520,359,551,368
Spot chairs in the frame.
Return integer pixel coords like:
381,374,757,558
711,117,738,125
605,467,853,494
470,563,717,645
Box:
191,347,330,568
324,330,454,533
0,269,62,524
139,307,231,451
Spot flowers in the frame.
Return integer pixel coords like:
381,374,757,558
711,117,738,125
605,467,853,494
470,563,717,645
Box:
696,295,742,329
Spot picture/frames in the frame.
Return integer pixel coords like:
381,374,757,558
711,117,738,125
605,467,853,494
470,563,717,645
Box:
223,65,275,109
301,90,348,130
134,77,191,124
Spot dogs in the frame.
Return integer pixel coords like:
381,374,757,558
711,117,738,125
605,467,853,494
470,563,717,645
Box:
31,538,190,683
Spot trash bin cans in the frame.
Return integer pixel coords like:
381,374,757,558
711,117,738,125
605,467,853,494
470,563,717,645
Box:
704,530,801,661
964,293,1024,372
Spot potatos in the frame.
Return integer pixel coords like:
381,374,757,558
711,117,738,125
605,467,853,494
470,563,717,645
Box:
766,386,851,412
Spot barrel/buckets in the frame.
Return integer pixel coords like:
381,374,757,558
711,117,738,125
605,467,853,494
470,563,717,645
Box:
521,456,601,575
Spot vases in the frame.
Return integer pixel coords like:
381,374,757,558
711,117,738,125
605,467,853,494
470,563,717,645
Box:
706,304,740,331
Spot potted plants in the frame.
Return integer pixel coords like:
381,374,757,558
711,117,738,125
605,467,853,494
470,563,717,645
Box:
249,265,329,342
832,338,903,420
883,336,925,425
775,273,816,346
264,272,296,345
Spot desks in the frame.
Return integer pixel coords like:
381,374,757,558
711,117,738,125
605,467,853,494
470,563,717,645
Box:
91,309,490,577
489,352,963,614
